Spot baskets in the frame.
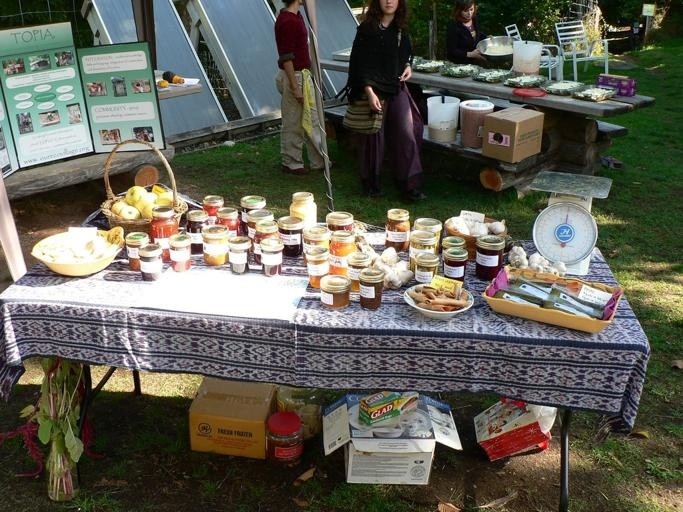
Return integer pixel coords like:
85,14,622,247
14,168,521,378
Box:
100,140,188,235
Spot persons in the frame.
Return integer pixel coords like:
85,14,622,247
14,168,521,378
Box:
349,0,428,201
447,0,493,64
275,0,342,175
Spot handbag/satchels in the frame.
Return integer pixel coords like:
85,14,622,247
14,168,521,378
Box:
342,99,385,135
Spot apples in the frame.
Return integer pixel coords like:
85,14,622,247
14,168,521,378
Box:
110,184,171,219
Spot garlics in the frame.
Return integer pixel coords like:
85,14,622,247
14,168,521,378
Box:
508,245,568,276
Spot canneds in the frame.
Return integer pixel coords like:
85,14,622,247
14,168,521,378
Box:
125,191,506,311
268,411,305,471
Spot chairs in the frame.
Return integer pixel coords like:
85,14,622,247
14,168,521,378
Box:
552,18,610,82
504,22,566,82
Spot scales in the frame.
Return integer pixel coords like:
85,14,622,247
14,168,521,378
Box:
528,171,613,275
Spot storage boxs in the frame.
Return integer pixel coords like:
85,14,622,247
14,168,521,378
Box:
186,375,279,463
481,105,546,164
320,392,465,490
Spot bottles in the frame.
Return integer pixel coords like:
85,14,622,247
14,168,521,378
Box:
154,70,184,87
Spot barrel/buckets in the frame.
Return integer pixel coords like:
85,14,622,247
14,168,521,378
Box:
426,96,459,142
512,39,544,78
460,100,494,148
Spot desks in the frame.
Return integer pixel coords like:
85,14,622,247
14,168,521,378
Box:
1,210,652,511
153,69,204,100
318,55,658,198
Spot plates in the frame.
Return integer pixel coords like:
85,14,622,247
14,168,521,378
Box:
14,85,75,110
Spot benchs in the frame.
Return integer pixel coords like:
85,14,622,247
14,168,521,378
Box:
2,142,176,200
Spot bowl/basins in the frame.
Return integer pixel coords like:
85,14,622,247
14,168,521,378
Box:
404,284,474,321
475,35,521,61
31,226,126,276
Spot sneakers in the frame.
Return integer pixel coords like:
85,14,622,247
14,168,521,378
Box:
401,189,425,201
281,163,309,175
365,187,385,197
